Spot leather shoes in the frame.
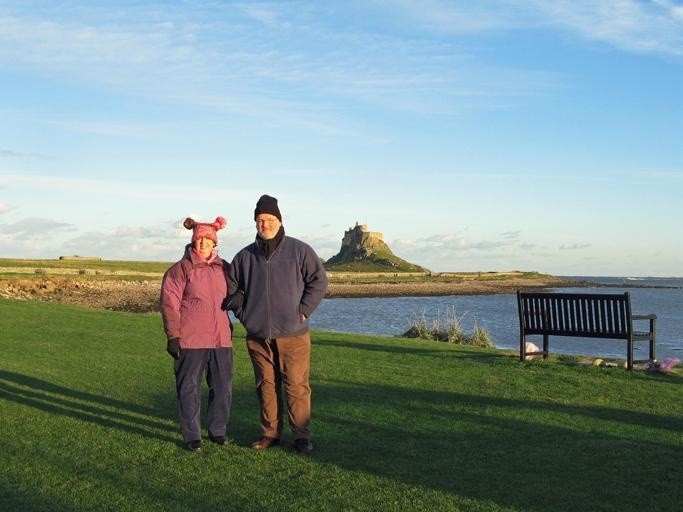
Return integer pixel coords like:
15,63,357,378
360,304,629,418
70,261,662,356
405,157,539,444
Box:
214,436,228,447
249,437,276,450
186,440,201,453
295,437,314,454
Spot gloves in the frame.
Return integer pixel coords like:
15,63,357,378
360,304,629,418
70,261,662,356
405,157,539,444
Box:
220,289,246,312
166,338,181,360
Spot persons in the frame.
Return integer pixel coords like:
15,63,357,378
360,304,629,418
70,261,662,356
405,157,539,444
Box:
161,216,245,451
228,195,328,454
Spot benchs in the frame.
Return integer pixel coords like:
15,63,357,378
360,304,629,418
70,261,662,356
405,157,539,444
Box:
516,288,658,371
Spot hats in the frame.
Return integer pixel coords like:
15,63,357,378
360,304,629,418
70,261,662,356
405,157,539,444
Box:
253,194,283,224
183,216,227,248
